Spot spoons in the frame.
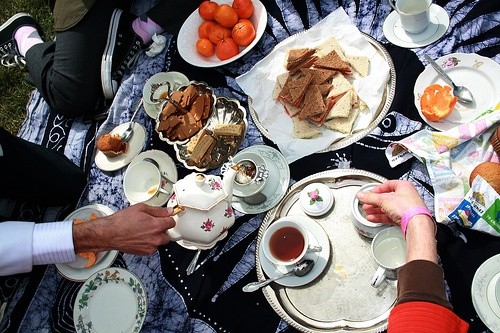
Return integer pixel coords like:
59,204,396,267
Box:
143,158,176,187
424,53,475,104
242,260,314,292
120,99,143,142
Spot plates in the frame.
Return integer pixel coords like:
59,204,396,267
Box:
74,267,147,333
54,203,119,281
95,122,145,171
223,145,291,215
143,70,190,120
413,53,500,133
383,4,449,48
125,150,177,206
470,254,500,333
259,215,331,287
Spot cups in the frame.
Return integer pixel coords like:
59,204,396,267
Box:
225,152,272,197
370,224,409,288
389,0,432,34
123,161,170,203
261,221,323,266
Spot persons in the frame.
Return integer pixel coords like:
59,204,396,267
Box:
0,0,209,120
356,180,469,333
0,127,175,276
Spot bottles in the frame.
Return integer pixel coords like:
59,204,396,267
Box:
351,183,390,238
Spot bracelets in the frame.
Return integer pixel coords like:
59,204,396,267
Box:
401,206,437,241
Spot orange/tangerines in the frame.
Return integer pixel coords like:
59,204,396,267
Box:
197,0,257,62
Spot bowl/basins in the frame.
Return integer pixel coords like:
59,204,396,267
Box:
177,0,267,68
143,72,174,105
63,208,106,270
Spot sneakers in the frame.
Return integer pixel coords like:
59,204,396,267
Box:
103,8,150,99
0,11,41,71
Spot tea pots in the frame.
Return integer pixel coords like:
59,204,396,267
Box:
163,166,240,250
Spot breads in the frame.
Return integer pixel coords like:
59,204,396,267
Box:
273,37,370,138
468,161,500,196
491,125,500,155
186,122,242,163
157,85,211,140
97,134,127,157
